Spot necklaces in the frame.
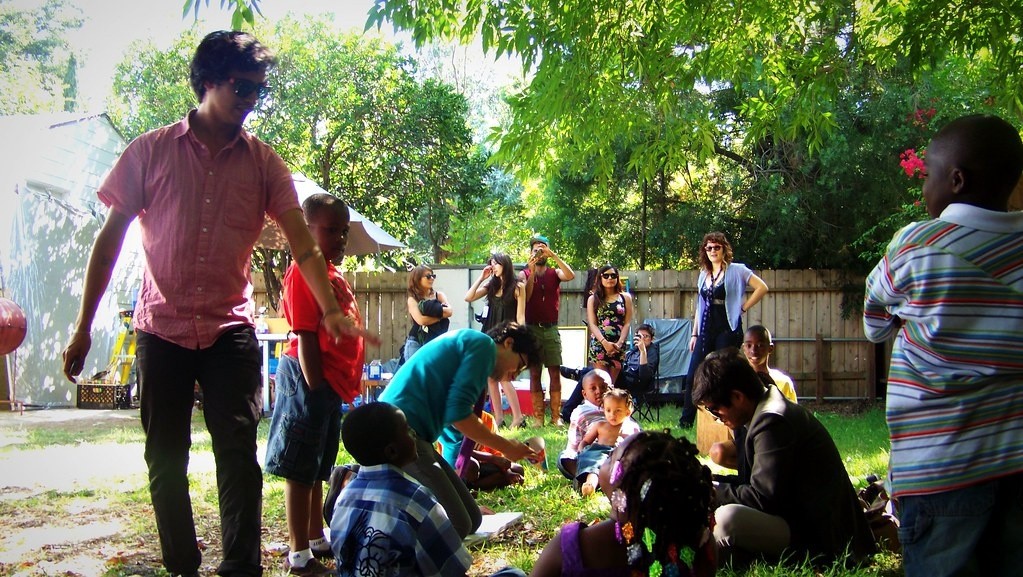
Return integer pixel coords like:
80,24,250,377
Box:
421,292,432,302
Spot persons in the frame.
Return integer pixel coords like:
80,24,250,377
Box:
691,350,881,572
584,266,633,388
862,115,1023,577
558,369,641,496
332,401,527,577
710,325,798,470
465,254,527,430
324,322,540,541
680,233,767,425
561,324,659,424
518,236,576,429
404,265,451,360
531,430,719,577
260,197,355,577
64,29,382,577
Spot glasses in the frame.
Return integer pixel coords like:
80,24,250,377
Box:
214,76,271,99
705,245,722,251
601,273,618,279
519,352,528,372
421,274,436,279
705,403,725,418
643,335,652,340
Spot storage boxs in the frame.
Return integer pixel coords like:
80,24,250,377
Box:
76,382,133,410
488,380,546,417
367,359,381,380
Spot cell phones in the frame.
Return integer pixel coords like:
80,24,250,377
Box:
534,250,545,258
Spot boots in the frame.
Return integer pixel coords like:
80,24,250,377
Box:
549,391,561,426
531,391,545,428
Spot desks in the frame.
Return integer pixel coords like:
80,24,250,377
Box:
361,379,389,404
256,331,296,417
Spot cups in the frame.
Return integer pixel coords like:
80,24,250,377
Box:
366,359,382,380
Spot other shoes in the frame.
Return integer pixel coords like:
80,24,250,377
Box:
284,557,337,577
323,465,354,529
508,420,526,430
497,420,506,429
674,421,693,429
311,546,335,558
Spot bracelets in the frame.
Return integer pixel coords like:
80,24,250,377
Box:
322,309,345,322
740,307,748,314
691,333,699,340
437,316,442,321
597,336,607,345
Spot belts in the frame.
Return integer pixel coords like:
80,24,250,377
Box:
526,321,557,328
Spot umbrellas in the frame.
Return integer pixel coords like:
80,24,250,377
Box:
250,167,413,259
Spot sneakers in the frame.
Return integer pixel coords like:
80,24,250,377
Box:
560,366,578,381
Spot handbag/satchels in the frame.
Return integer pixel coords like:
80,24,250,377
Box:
858,482,901,552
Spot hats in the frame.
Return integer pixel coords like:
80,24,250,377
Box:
530,236,549,246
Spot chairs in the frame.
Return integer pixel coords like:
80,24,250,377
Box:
617,342,660,423
